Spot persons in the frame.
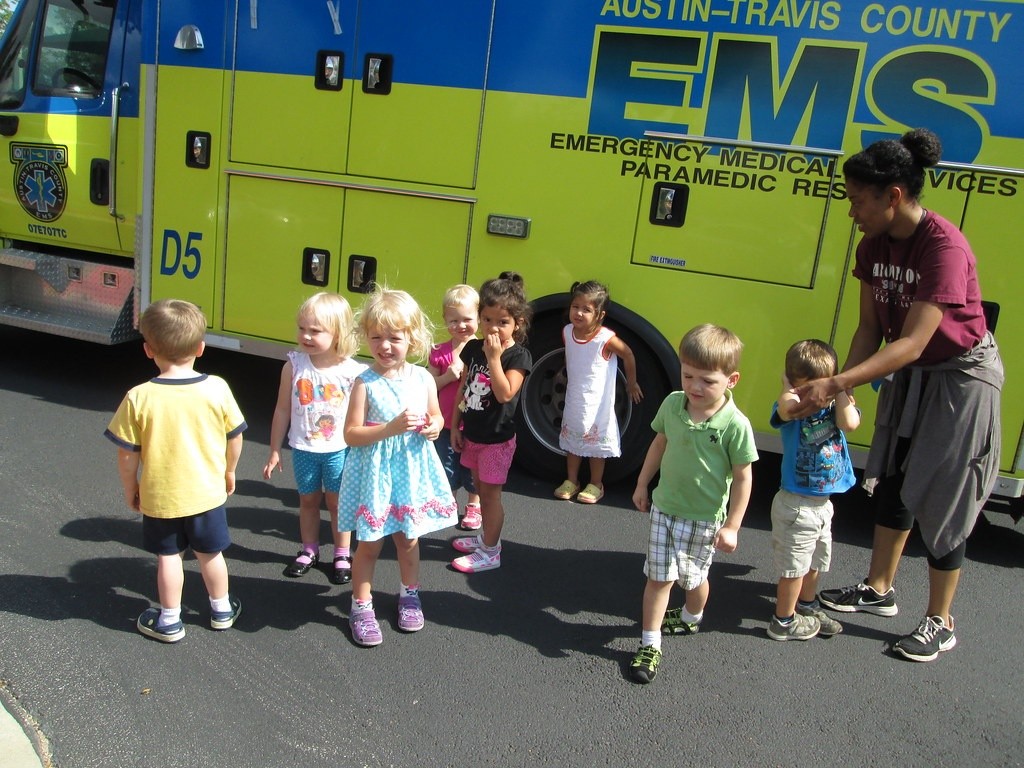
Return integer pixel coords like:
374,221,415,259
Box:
104,297,247,642
262,292,368,584
765,339,862,641
553,278,644,505
343,288,459,647
452,269,532,573
426,285,484,530
624,323,759,684
788,126,1004,664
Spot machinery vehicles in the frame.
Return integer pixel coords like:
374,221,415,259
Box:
4,0,1024,512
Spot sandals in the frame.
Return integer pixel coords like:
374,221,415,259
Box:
136,607,186,643
211,597,242,629
577,483,605,504
553,480,580,500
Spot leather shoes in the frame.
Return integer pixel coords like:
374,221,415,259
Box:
332,556,352,583
289,549,319,576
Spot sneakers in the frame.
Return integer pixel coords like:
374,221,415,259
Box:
629,644,662,682
452,547,500,573
893,615,957,661
348,608,383,646
818,578,898,616
460,503,482,530
796,594,842,635
767,610,820,641
398,596,423,631
452,533,502,552
660,607,703,635
